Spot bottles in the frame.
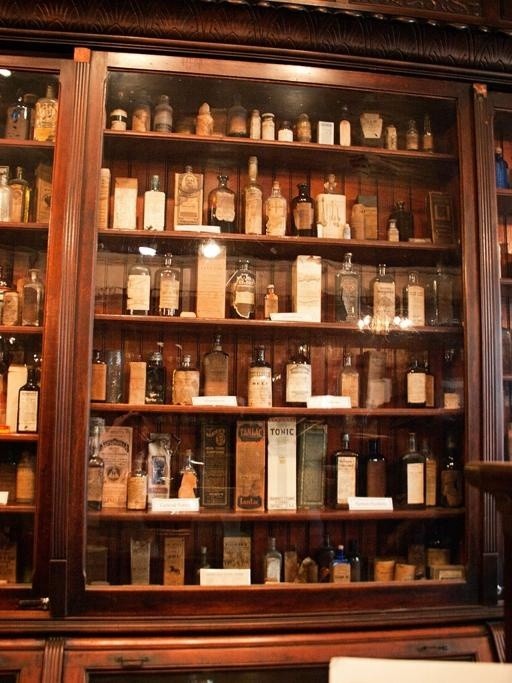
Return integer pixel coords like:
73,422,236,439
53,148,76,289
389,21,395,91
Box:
371,263,396,323
0,335,39,434
0,263,45,327
247,344,275,409
104,82,440,154
330,429,460,506
86,426,201,510
0,160,32,223
203,333,230,403
198,531,455,583
147,352,168,404
228,257,256,320
283,343,312,408
262,283,280,320
402,271,426,326
93,350,122,402
338,349,360,409
13,447,34,505
174,353,200,407
334,252,362,323
143,155,415,241
3,80,59,141
494,146,510,190
402,350,436,409
125,250,183,316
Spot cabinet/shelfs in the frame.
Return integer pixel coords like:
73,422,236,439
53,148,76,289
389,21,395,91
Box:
0,619,512,681
63,48,478,623
0,44,78,618
471,80,512,614
